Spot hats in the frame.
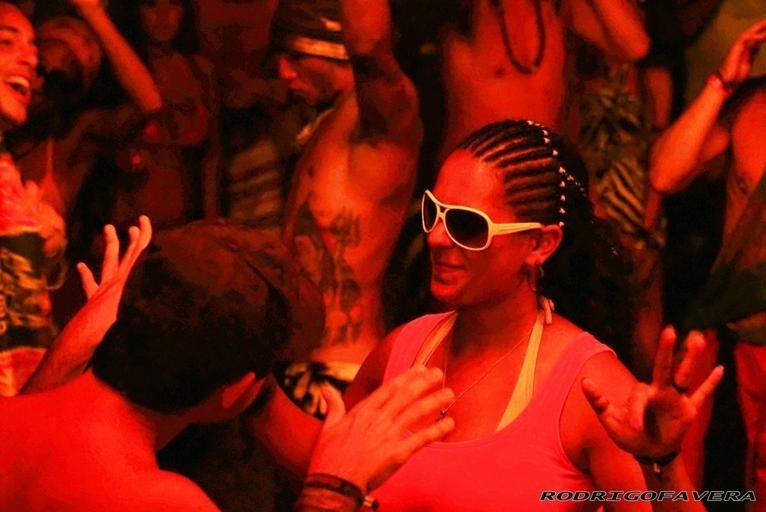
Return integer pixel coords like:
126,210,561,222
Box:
270,0,353,63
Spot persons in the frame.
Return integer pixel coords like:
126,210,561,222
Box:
2,1,766,510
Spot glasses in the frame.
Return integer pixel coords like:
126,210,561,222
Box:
419,188,546,253
